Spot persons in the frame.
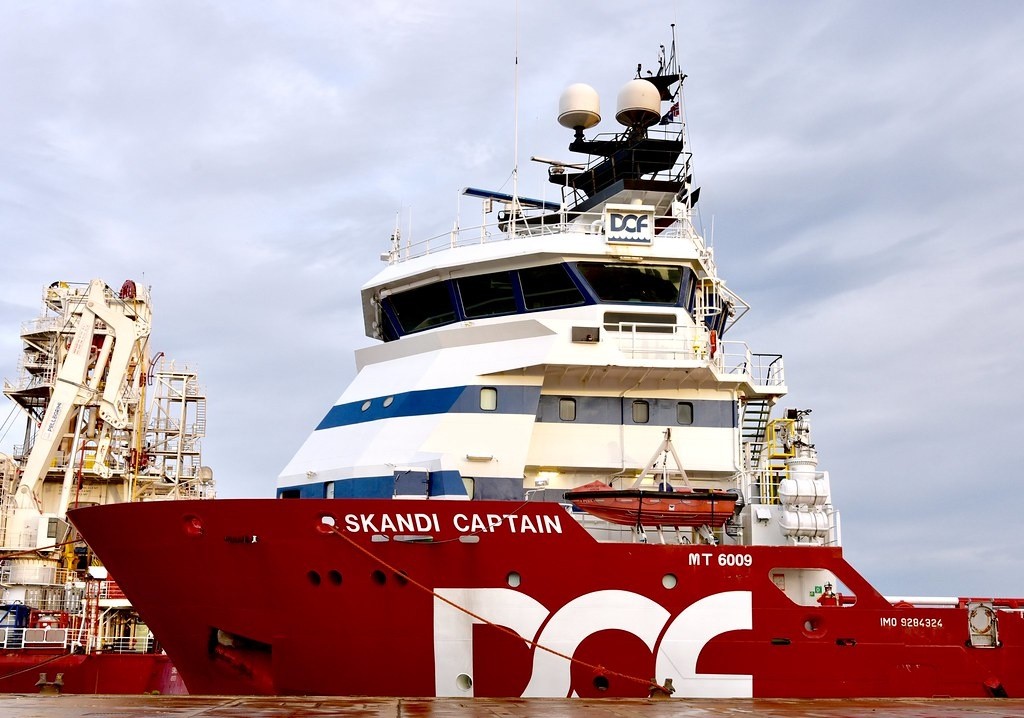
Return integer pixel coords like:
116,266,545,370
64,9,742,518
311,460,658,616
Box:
818,582,836,606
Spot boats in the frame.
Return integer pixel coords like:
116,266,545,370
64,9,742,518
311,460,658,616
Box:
569,427,735,527
1,268,221,697
67,19,1023,699
